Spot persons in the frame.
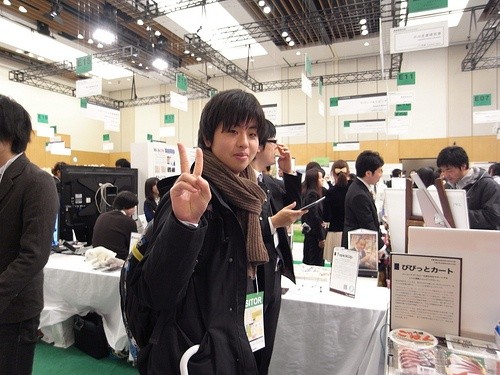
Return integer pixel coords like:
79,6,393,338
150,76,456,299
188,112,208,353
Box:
116,158,131,168
437,147,500,230
137,90,269,374
254,119,311,374
52,162,67,193
342,151,386,260
489,163,500,184
93,188,138,260
144,176,161,222
302,160,354,267
387,167,442,188
0,94,60,375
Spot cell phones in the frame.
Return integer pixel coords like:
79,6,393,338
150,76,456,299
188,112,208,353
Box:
300,195,326,211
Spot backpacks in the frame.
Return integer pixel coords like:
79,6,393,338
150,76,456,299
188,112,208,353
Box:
118,220,155,360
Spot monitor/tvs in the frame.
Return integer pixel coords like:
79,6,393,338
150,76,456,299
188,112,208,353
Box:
57,164,139,246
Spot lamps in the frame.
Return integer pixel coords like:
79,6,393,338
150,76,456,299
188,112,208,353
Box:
50,0,63,17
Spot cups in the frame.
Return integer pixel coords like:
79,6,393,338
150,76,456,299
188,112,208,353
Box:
387,327,439,375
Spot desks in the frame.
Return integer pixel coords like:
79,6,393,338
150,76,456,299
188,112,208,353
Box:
39,251,390,375
